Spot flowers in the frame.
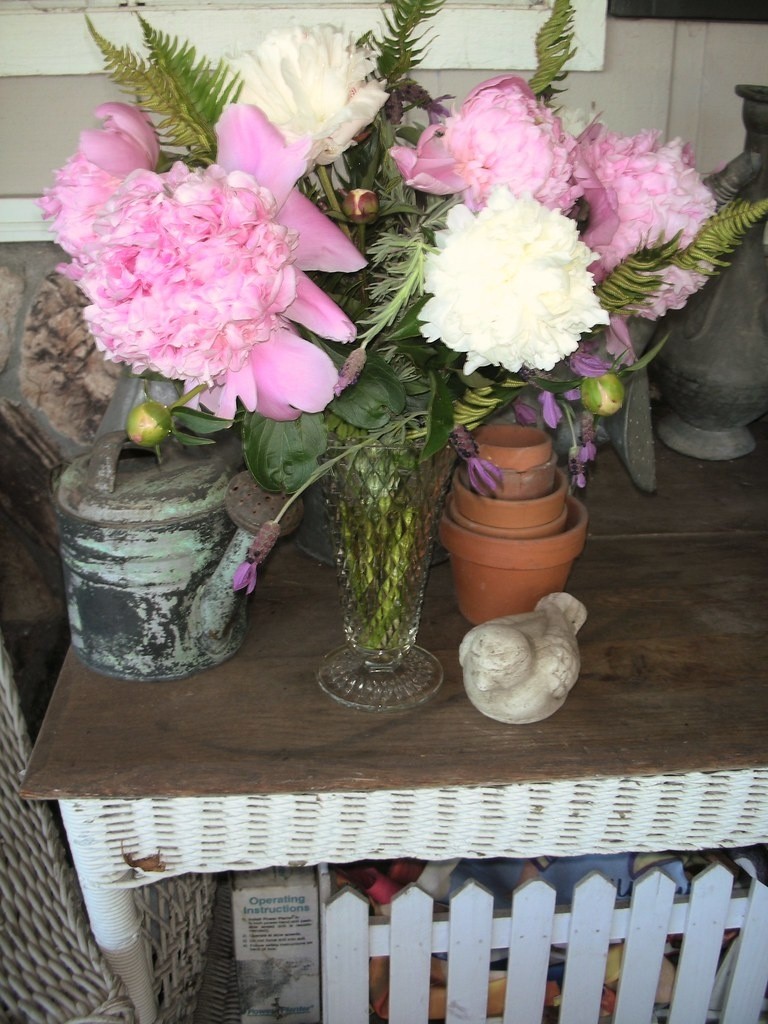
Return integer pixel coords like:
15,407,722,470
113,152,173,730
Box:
38,0,767,656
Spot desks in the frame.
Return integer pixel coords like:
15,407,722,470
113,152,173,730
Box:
21,402,768,1024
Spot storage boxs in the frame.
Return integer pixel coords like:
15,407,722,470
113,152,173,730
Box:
227,868,322,1024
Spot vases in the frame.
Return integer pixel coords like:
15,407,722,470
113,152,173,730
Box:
475,450,558,500
438,491,589,626
448,497,568,540
647,85,767,460
476,425,553,472
452,465,569,528
316,432,451,711
